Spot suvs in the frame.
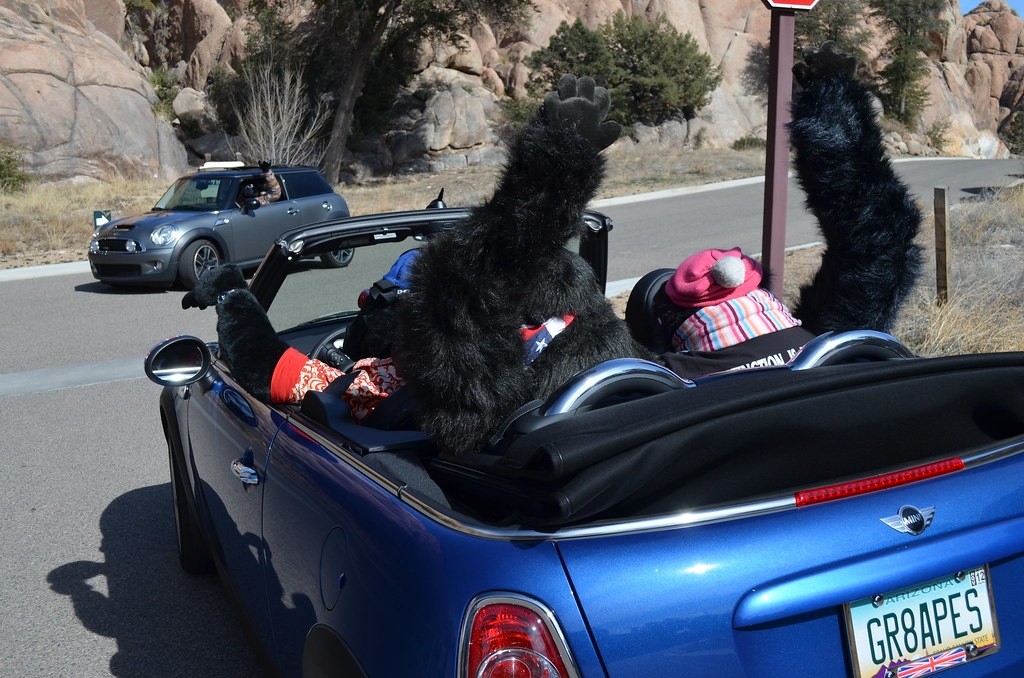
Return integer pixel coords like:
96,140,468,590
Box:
88,161,356,292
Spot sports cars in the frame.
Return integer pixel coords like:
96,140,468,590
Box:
144,188,1024,678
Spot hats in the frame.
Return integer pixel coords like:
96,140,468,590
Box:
666,245,763,307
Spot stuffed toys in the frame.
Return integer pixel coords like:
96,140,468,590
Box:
182,248,423,420
241,160,281,204
388,51,924,450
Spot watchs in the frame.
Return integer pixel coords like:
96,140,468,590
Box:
217,289,234,304
